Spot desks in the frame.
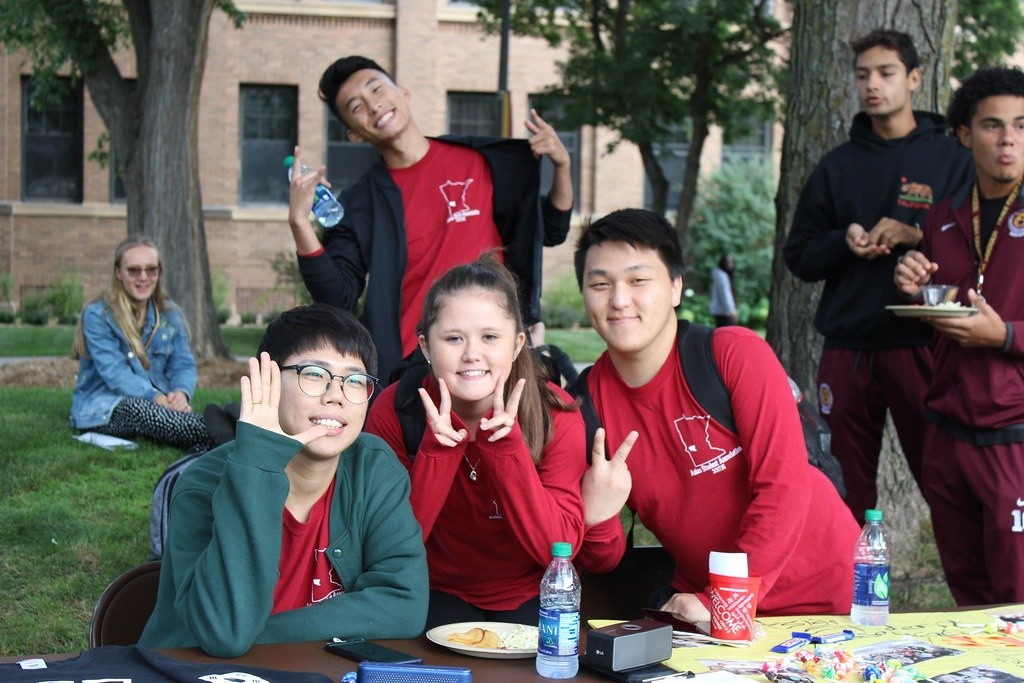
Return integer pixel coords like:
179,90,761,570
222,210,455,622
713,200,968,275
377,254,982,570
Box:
0,601,1024,683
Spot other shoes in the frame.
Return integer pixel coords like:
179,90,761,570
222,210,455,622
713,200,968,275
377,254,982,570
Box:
204,403,234,447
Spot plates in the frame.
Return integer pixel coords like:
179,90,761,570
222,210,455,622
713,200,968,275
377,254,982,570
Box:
885,305,978,317
426,621,539,659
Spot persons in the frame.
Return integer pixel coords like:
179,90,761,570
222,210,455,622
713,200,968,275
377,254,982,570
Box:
783,28,997,611
365,246,586,632
68,238,238,456
709,251,737,329
288,54,577,363
892,63,1024,606
559,207,872,623
135,307,432,655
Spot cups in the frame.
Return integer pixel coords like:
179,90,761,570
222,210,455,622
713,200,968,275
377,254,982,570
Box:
708,573,762,641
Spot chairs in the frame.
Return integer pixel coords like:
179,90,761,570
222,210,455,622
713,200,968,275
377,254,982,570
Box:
578,546,676,628
87,560,161,648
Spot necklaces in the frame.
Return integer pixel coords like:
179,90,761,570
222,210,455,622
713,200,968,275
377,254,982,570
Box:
461,452,482,481
143,295,160,352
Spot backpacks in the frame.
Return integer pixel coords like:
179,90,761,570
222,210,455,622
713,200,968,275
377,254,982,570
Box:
564,321,848,502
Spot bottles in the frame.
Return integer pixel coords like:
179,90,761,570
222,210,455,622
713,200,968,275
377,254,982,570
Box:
282,156,345,227
850,509,890,627
536,543,582,678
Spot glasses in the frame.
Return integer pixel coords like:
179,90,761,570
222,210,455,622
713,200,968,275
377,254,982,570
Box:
118,266,160,278
276,365,380,405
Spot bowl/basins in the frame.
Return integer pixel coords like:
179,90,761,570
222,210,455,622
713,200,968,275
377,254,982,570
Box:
924,285,958,306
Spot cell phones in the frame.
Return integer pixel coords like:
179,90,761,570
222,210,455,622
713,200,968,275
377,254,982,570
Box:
326,639,423,666
